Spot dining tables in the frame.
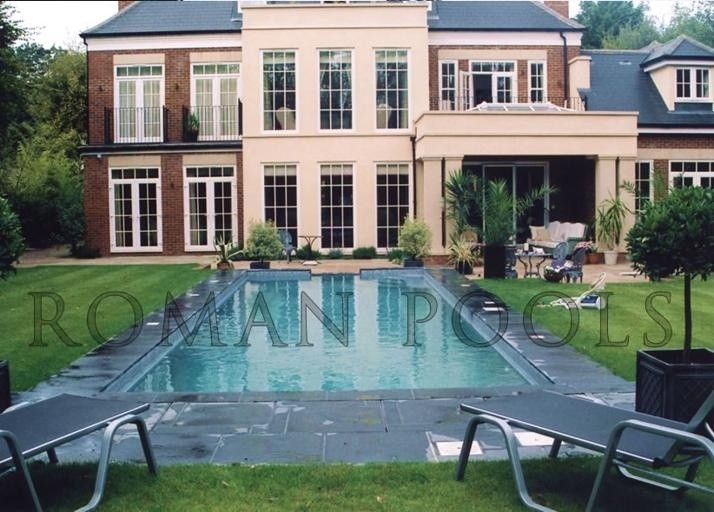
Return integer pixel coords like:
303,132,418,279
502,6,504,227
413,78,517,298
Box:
624,183,714,443
585,191,637,264
209,217,287,268
181,111,202,143
396,168,558,280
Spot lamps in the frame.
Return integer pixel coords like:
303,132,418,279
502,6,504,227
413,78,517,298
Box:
544,240,589,283
455,389,714,508
4,356,158,507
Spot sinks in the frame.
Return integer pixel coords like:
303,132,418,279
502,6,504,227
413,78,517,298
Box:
523,219,590,259
